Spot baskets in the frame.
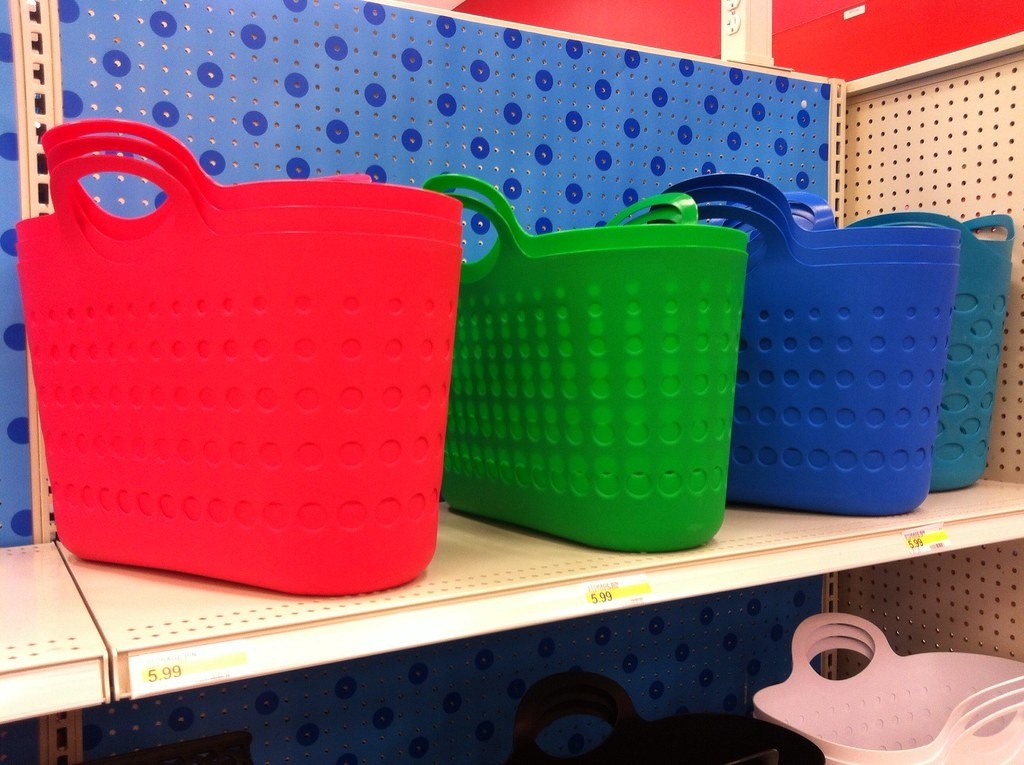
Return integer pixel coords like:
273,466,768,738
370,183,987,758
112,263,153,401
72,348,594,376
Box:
412,173,748,554
13,116,464,599
646,174,964,518
751,612,1024,765
834,210,1015,492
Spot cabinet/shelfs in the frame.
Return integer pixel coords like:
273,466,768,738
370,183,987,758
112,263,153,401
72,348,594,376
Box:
0,0,1024,765
